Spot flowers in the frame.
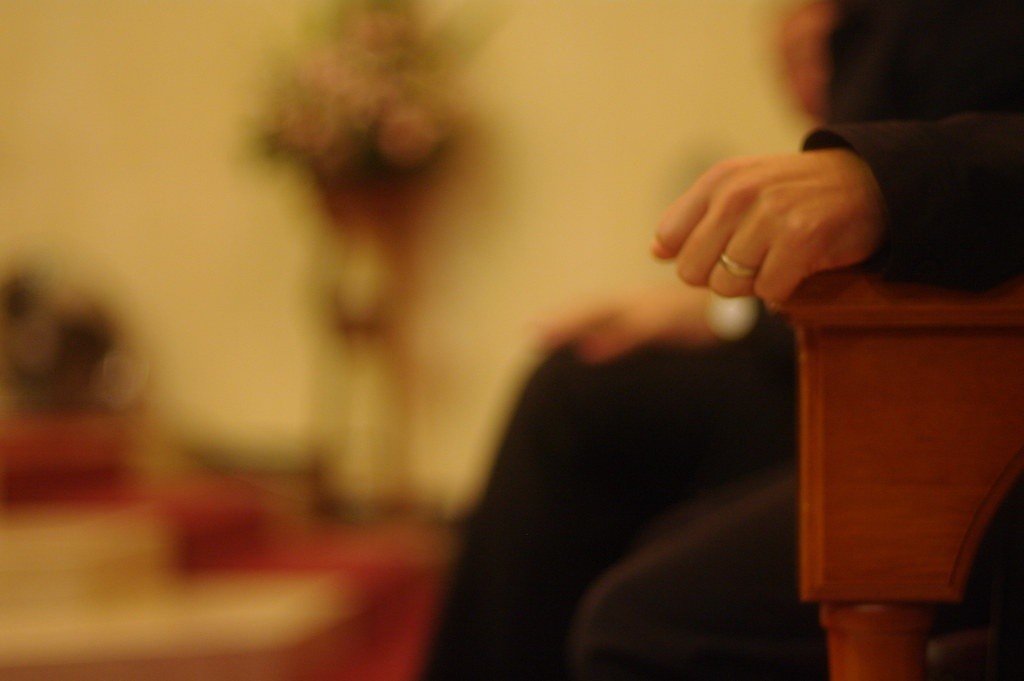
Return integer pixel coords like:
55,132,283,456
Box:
236,11,476,180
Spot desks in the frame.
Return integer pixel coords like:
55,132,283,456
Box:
0,525,474,679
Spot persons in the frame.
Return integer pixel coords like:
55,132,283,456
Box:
413,0,1024,681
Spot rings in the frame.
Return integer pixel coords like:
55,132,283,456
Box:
720,256,758,278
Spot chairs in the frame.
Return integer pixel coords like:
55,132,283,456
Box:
780,270,1024,681
559,469,999,680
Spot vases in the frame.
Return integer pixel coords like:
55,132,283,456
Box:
311,183,412,525
